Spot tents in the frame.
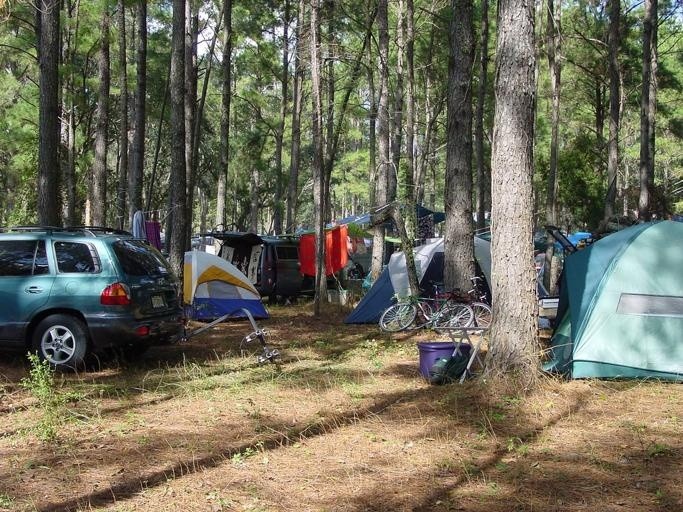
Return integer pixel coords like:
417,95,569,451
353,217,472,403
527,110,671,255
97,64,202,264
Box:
166,251,270,321
343,233,548,324
542,218,683,381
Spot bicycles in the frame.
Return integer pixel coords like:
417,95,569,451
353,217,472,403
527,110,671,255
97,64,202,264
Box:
379,276,474,335
396,277,492,337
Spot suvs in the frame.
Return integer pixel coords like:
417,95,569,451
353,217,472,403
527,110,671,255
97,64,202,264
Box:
0,226,184,371
198,231,342,298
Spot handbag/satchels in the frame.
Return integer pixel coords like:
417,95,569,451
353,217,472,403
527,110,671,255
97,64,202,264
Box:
427,355,469,385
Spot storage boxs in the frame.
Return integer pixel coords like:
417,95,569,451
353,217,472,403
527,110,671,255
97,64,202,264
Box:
417,342,472,380
